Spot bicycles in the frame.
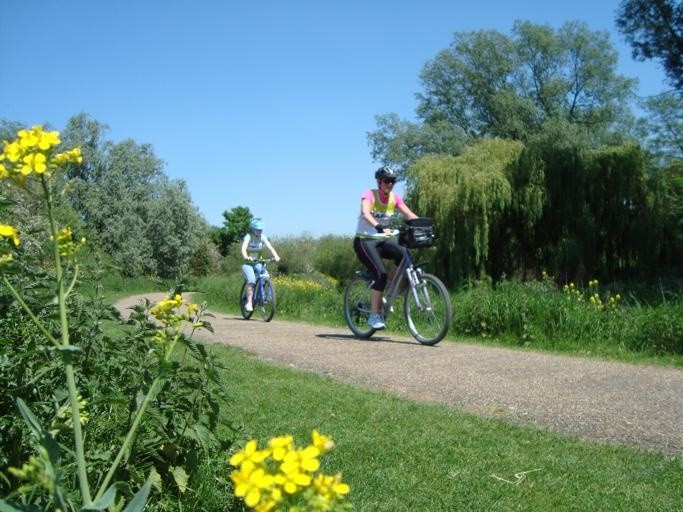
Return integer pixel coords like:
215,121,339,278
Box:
342,223,452,346
239,256,280,321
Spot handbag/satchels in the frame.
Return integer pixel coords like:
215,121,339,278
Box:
398,217,436,248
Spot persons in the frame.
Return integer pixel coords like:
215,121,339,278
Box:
353,166,420,330
241,217,280,312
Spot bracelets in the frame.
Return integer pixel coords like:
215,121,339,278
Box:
373,223,387,233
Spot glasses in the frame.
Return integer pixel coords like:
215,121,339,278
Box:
383,179,396,184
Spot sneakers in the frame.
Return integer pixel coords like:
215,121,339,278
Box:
366,313,386,329
244,304,253,312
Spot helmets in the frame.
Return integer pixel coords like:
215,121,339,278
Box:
250,217,263,230
374,166,397,179
